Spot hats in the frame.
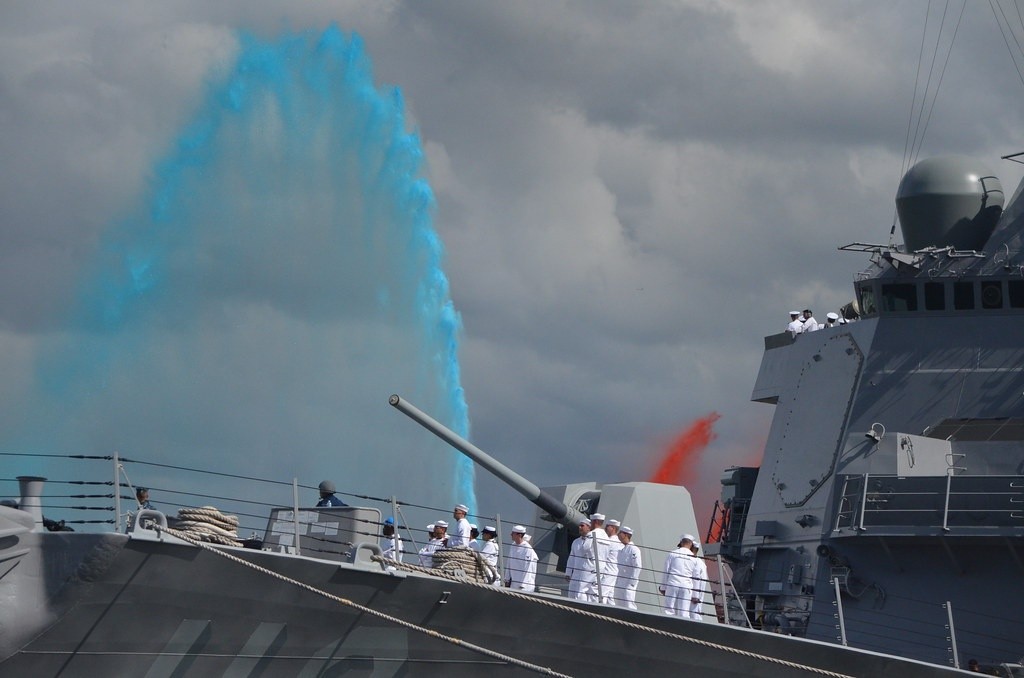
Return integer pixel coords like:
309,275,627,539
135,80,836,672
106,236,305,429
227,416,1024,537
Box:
435,520,449,527
317,480,336,493
788,311,800,315
620,526,634,535
838,317,849,323
693,541,700,549
676,534,695,547
606,519,620,527
469,524,477,528
455,504,469,512
481,525,496,532
827,312,838,320
427,524,435,532
590,513,605,520
512,525,526,533
580,519,591,527
384,517,400,527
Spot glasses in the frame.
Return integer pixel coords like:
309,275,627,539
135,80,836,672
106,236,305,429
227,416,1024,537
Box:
579,525,584,528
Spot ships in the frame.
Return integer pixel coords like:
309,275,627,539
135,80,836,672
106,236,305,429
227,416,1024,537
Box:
0,1,1023,678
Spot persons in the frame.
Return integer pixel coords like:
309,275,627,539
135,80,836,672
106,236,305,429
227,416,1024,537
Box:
503,525,540,593
658,534,707,622
614,525,642,610
565,513,625,611
383,517,404,563
136,486,158,511
418,504,500,586
316,480,350,507
785,309,850,335
